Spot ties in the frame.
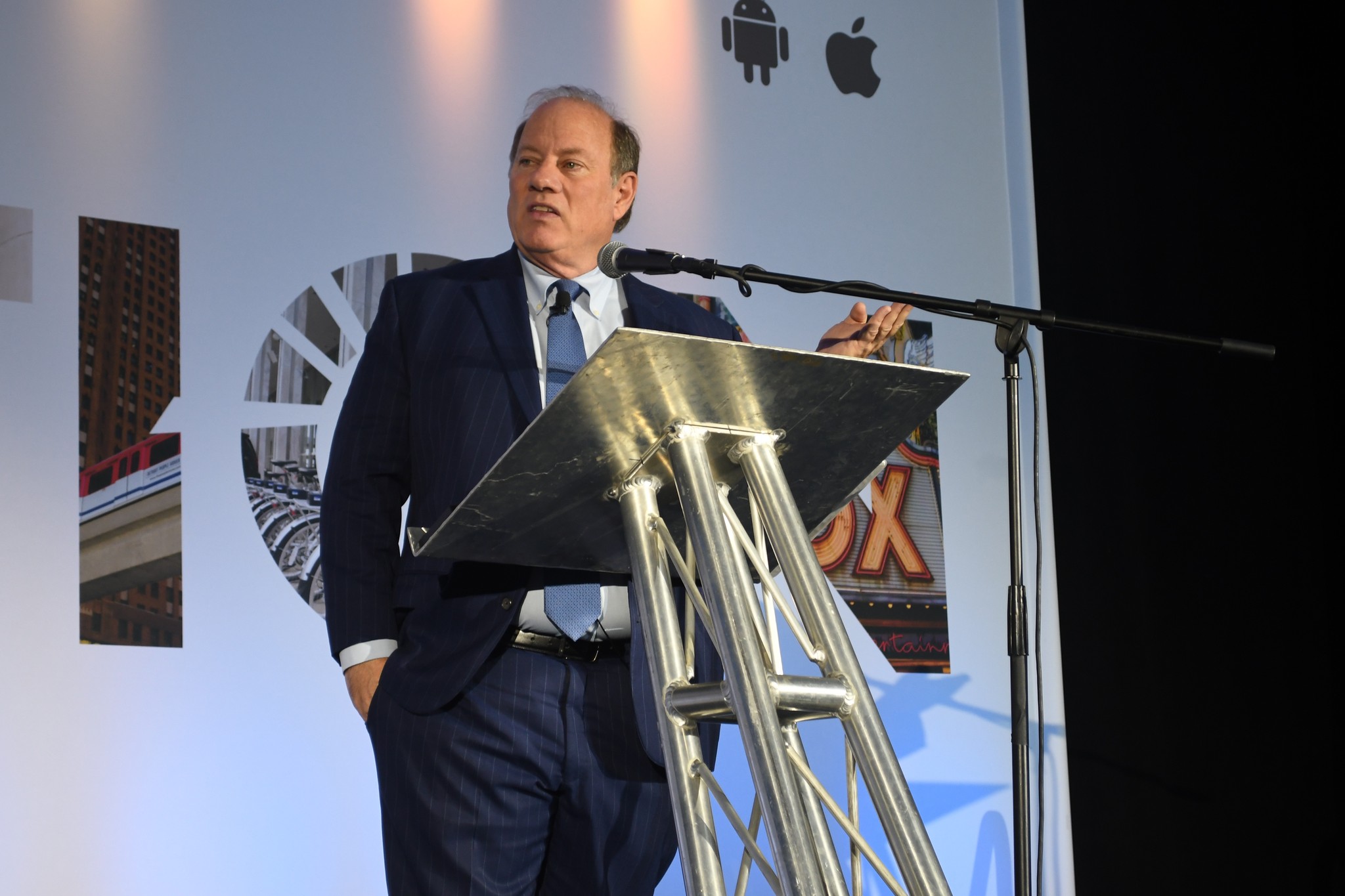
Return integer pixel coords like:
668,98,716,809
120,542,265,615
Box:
541,278,603,644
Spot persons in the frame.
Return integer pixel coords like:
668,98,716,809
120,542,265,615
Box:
321,85,914,896
882,319,918,367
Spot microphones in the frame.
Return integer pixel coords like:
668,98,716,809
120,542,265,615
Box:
596,241,705,280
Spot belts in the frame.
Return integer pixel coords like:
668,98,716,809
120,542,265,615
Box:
502,628,628,663
